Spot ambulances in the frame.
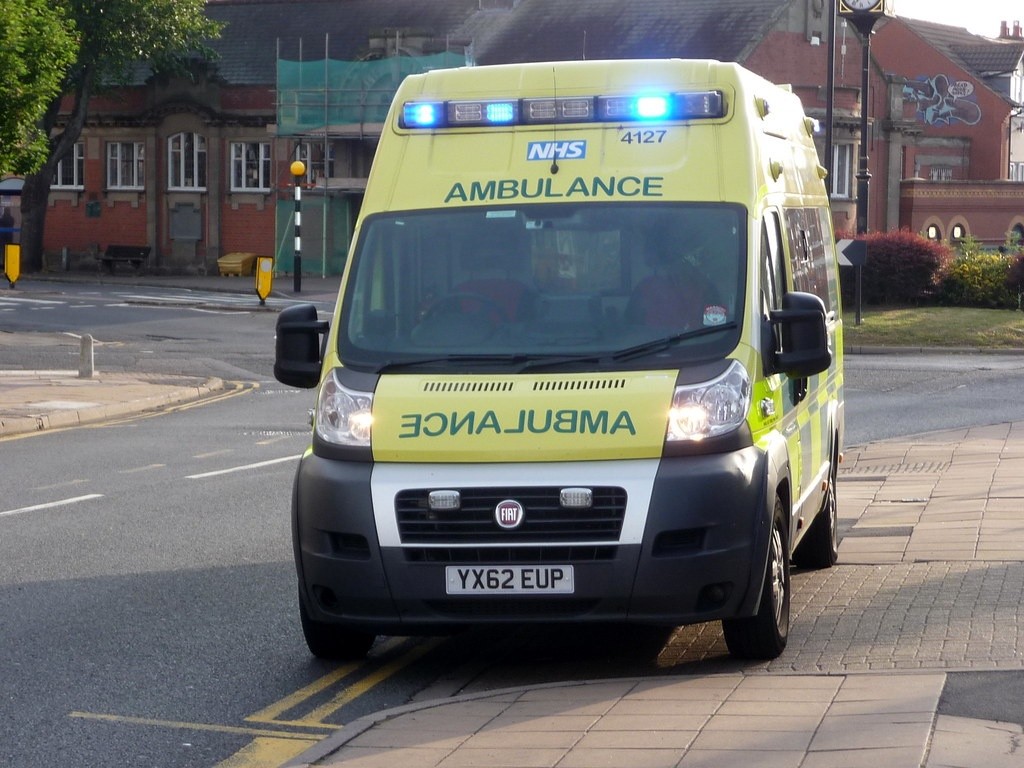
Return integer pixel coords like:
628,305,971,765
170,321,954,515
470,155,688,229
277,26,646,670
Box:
273,55,849,660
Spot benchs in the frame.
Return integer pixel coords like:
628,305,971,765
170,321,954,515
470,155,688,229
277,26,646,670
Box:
95,245,151,273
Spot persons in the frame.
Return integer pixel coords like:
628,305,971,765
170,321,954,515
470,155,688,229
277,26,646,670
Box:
0,206,14,271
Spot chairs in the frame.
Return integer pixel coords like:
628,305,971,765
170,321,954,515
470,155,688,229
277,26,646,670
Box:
624,215,721,329
451,228,530,323
